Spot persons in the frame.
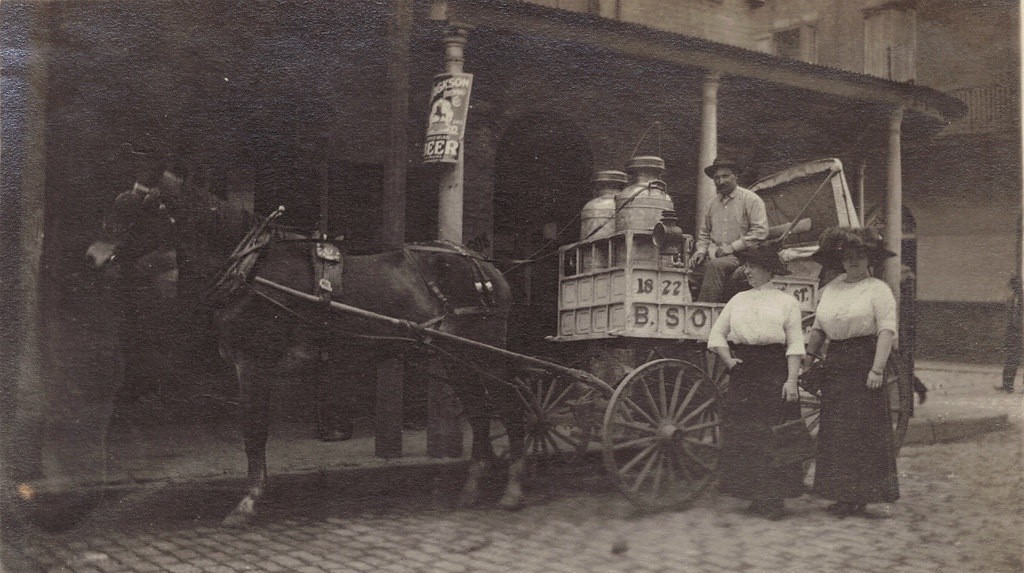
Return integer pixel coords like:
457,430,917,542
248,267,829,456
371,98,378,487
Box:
707,244,810,522
995,275,1023,395
799,224,898,518
687,153,770,305
913,374,928,405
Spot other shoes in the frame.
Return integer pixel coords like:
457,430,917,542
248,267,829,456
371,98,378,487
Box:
918,384,927,404
745,496,784,519
827,499,867,518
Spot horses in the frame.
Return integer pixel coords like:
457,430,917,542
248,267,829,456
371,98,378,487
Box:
85,170,528,530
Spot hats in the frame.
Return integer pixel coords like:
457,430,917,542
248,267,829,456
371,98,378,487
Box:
733,245,792,276
811,226,898,264
705,155,743,177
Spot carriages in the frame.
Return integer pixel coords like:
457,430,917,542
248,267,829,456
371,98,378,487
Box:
84,148,932,517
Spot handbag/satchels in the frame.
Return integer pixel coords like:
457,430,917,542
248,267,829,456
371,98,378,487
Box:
798,352,825,396
771,418,819,467
